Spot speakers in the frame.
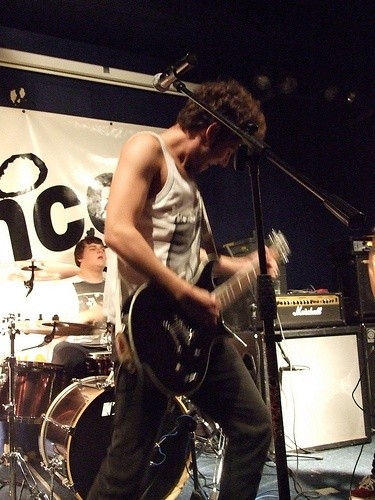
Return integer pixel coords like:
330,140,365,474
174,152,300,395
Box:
231,323,371,462
333,252,375,324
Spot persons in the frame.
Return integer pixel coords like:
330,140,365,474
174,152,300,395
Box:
84,83,281,499
49,236,107,365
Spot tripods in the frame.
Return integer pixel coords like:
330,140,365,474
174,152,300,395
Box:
0,313,50,499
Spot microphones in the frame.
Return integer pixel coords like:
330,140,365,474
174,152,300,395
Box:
151,51,198,91
279,367,302,372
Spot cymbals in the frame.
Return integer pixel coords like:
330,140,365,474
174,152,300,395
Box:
12,320,106,336
0,260,81,282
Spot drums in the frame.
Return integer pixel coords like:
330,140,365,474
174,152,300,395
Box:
0,357,74,425
38,375,196,500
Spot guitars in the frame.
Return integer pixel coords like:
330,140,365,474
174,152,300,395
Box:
114,227,292,398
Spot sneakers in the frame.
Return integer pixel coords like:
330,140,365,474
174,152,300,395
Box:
350,475,375,500
